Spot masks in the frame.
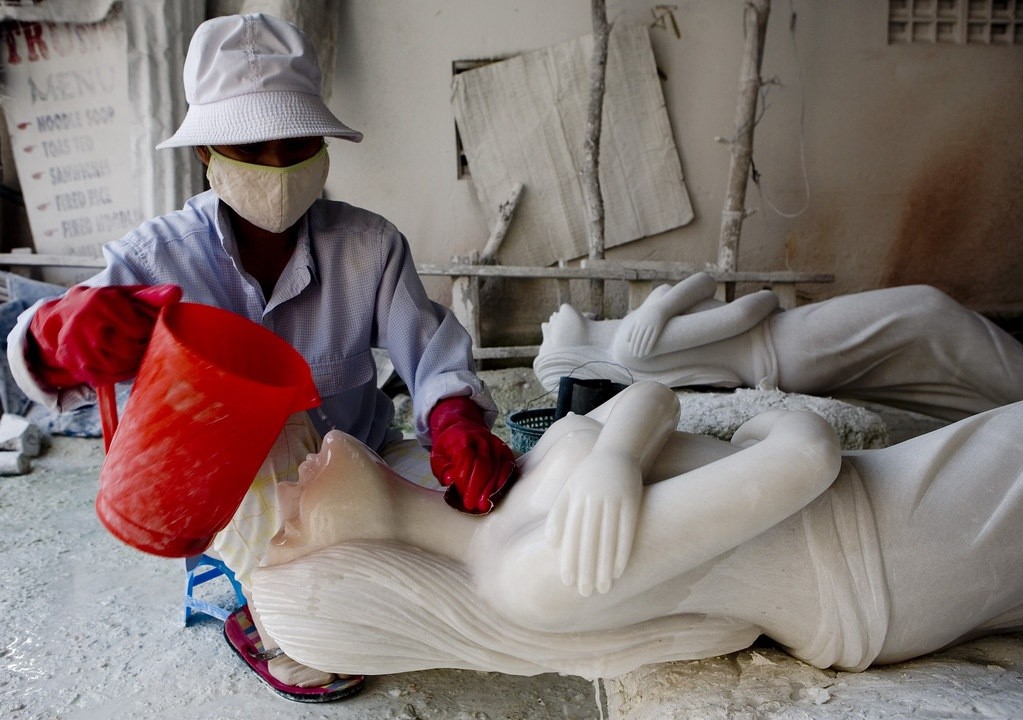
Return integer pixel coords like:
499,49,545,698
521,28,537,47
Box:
205,145,330,234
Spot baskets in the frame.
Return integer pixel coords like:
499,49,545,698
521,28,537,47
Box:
506,360,634,455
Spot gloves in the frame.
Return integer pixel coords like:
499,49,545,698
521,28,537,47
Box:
28,284,184,387
428,396,513,513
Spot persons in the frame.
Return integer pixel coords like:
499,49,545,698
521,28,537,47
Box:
0,12,517,702
533,272,1023,422
249,379,1023,680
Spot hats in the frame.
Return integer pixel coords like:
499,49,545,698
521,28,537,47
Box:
155,13,364,151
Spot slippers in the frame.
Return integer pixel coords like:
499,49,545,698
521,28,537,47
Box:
223,604,366,704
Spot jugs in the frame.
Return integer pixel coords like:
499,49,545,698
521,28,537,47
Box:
96,300,321,557
